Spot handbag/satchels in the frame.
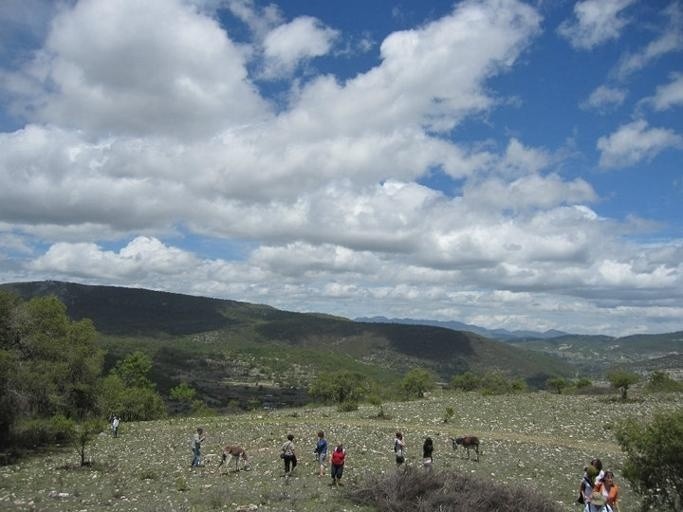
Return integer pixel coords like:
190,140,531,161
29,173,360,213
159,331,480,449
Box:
281,453,285,458
578,495,584,504
396,456,404,462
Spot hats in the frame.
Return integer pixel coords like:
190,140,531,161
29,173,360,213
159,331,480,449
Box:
588,491,607,505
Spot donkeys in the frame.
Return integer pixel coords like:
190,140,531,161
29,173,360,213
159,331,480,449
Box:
448,436,480,464
214,445,248,473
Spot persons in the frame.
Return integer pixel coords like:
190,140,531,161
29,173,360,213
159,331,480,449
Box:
330,441,346,488
579,458,620,512
112,416,119,438
312,430,328,477
281,434,297,476
393,432,405,464
191,427,206,467
332,447,345,465
422,437,433,464
